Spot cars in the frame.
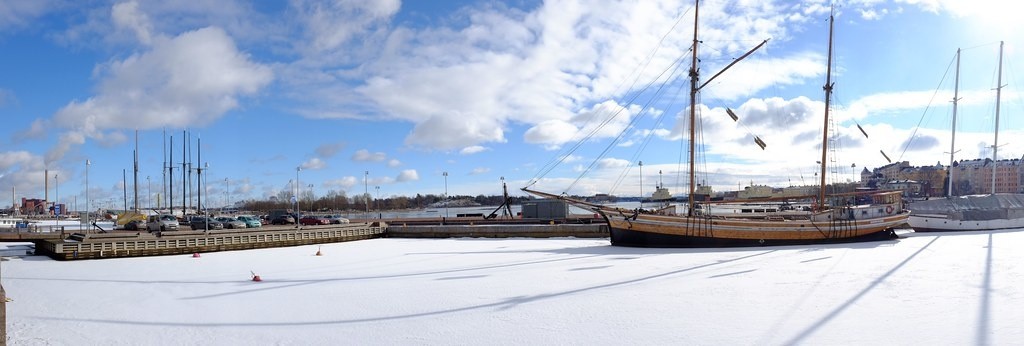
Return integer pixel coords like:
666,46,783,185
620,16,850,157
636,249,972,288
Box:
122,212,349,231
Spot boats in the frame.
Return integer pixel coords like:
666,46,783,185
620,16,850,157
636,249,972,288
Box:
638,169,677,203
684,179,725,202
732,179,817,201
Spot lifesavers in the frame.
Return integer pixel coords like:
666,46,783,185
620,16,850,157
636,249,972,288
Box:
886,206,893,214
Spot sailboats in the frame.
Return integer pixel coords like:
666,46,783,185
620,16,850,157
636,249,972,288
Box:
518,0,913,249
880,40,1024,232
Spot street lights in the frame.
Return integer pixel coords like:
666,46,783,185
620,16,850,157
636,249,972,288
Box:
850,163,857,206
295,166,302,228
442,172,449,218
374,186,381,220
364,170,369,224
203,162,210,231
500,176,505,217
638,160,644,210
85,159,91,227
307,183,315,215
